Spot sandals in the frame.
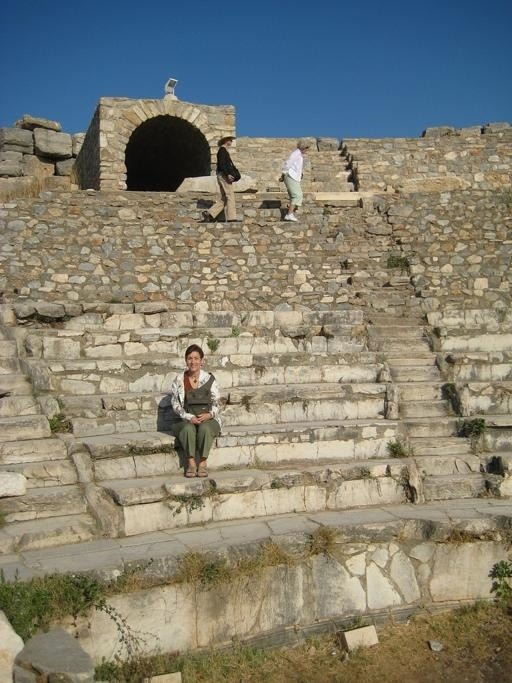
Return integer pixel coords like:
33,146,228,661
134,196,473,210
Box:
185,464,198,477
198,462,209,477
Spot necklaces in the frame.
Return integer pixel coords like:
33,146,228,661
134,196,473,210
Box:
192,376,199,384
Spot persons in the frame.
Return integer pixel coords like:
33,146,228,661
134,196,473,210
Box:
192,197,226,223
259,197,290,221
201,131,240,223
157,391,189,476
171,344,223,478
279,138,310,222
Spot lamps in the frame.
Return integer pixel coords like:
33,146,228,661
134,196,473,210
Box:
164,78,178,101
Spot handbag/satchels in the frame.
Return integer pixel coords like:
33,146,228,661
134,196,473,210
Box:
184,371,216,418
222,165,241,182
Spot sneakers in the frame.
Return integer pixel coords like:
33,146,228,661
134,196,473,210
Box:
284,213,297,222
227,219,243,222
200,210,212,223
